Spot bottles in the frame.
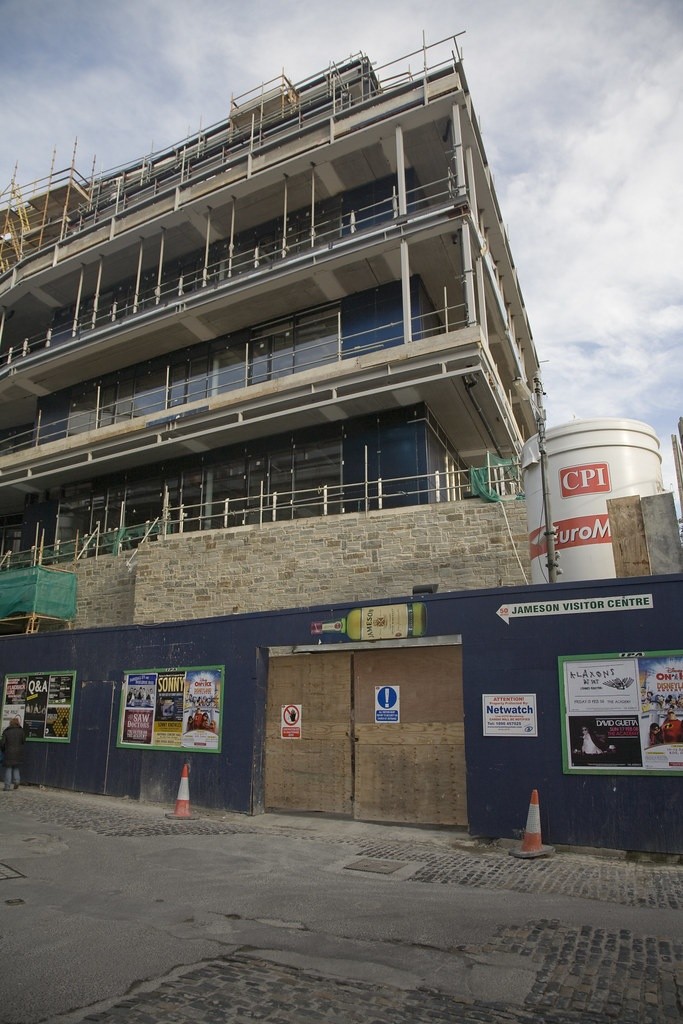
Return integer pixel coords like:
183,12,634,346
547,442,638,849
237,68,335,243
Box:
311,602,428,641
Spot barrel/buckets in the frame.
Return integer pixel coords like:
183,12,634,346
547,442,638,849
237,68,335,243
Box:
522,418,663,585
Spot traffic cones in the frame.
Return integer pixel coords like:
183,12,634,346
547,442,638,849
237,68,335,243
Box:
509,789,556,860
164,764,200,821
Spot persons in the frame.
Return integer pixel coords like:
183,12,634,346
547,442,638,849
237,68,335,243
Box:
0,718,26,791
572,724,616,767
642,692,683,746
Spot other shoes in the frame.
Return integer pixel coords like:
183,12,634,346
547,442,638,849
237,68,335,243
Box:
3,784,10,791
14,782,19,789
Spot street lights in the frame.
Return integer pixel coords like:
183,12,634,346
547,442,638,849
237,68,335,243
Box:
511,375,562,584
